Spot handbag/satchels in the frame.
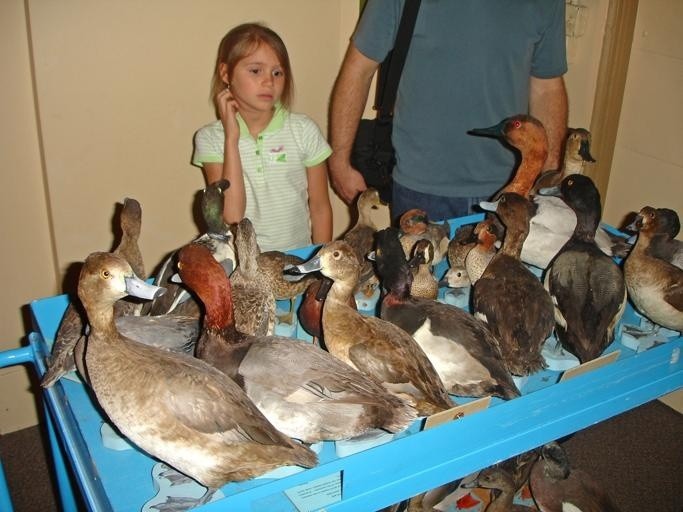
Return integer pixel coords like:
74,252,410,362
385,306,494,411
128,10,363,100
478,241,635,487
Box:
352,118,394,201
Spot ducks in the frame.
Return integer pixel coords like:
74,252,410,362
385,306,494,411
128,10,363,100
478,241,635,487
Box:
40,115,683,512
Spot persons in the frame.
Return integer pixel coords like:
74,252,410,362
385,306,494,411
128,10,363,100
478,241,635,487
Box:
191,20,334,253
326,0,571,230
